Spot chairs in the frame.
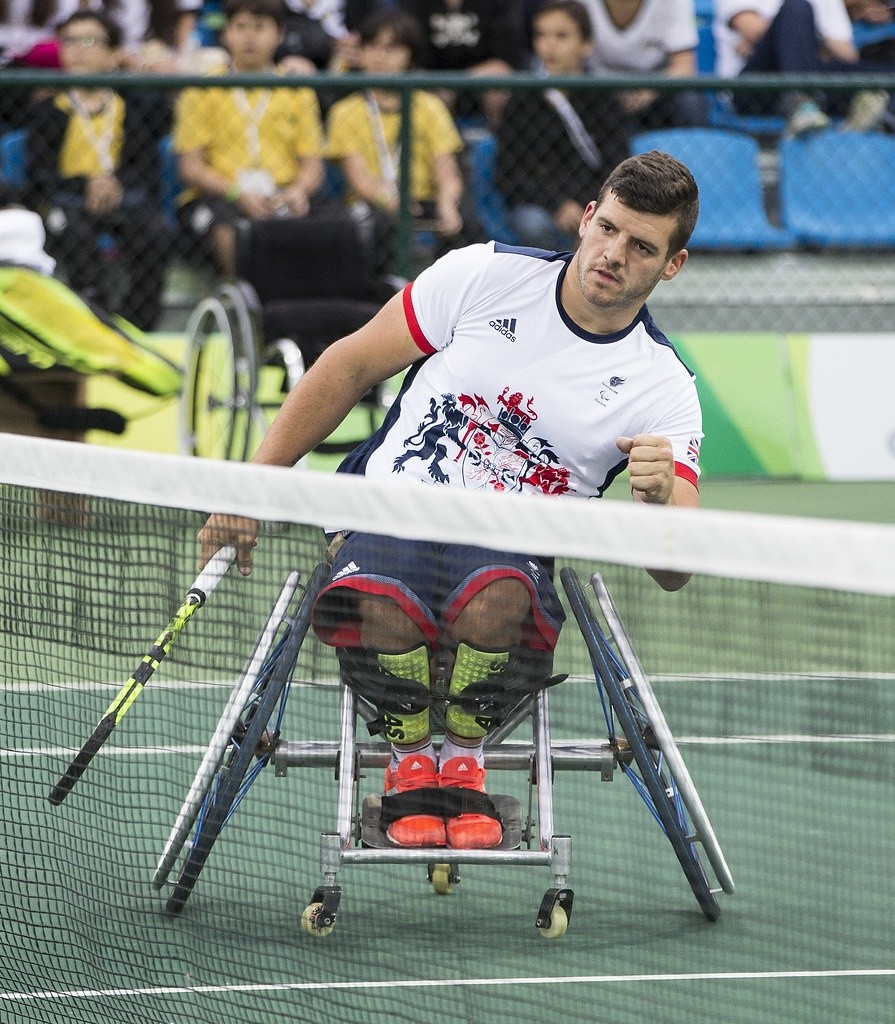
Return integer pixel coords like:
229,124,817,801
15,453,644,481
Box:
0,128,114,245
628,126,796,250
156,134,183,221
694,0,785,137
775,125,895,249
473,140,520,246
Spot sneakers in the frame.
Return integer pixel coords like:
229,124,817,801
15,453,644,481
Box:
437,756,503,849
384,753,446,848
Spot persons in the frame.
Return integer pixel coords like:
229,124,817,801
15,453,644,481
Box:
0,0,895,333
197,150,703,849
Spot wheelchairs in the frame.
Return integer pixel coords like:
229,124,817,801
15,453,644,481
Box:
177,215,449,461
152,562,736,939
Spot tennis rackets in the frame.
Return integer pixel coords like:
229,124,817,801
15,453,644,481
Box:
42,542,239,808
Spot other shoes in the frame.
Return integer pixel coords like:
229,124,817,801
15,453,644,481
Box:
791,104,833,134
